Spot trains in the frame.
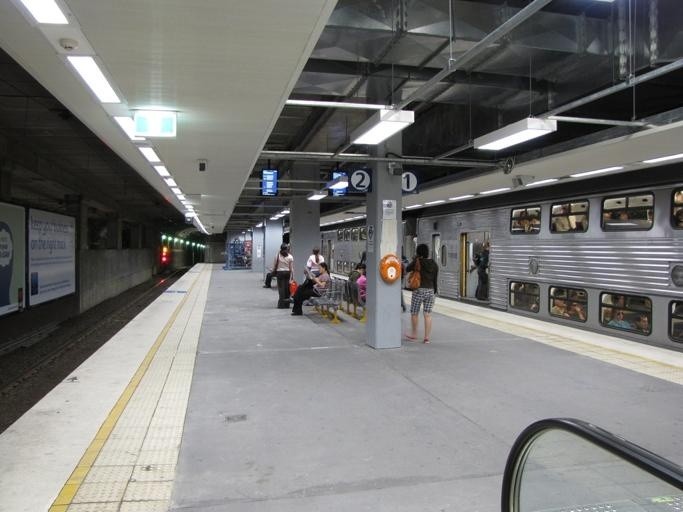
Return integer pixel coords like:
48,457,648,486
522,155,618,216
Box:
320,181,683,353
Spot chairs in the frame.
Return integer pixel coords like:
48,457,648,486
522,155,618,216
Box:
307,275,366,323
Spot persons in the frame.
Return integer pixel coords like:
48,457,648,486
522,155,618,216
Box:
507,279,682,345
403,244,438,344
272,242,294,308
349,264,365,282
399,255,410,312
508,192,683,234
470,238,490,300
306,247,325,277
356,272,367,303
284,263,329,315
262,270,277,287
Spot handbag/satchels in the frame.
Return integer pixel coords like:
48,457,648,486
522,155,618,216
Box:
406,271,421,289
290,281,297,295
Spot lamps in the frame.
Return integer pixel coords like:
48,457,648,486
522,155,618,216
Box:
348,1,415,145
467,18,557,152
242,189,328,234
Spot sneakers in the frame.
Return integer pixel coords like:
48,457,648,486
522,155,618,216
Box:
292,311,303,315
407,336,418,340
424,337,430,343
